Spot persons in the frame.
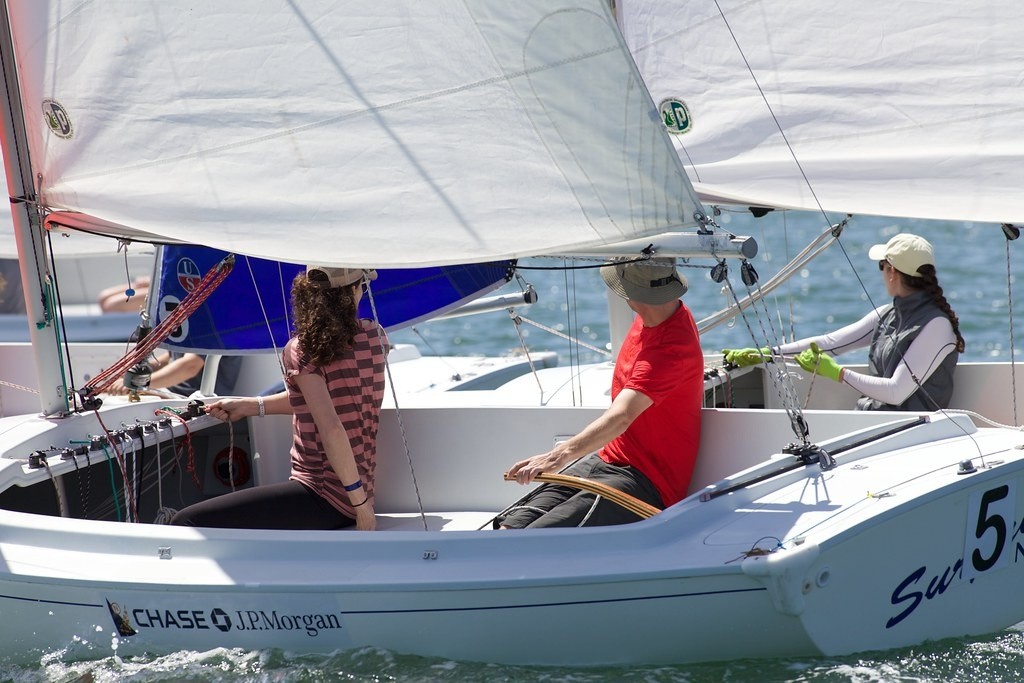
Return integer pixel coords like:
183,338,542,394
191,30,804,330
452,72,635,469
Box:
499,257,704,529
170,265,389,531
100,278,152,312
104,349,243,405
722,233,965,411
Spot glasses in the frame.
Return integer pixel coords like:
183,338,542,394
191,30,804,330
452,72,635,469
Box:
879,260,891,270
361,279,371,293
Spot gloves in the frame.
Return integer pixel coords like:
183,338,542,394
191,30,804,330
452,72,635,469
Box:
722,347,771,368
794,342,842,382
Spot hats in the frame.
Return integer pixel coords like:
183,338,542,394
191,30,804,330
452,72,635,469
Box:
599,256,688,304
868,233,936,277
305,265,378,289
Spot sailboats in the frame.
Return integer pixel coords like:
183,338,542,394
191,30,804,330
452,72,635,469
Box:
0,1,1024,673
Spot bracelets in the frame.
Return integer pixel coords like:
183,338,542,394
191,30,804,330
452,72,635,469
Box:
343,480,363,491
351,498,368,507
256,396,264,417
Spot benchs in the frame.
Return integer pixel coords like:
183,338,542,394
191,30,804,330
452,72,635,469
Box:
375,512,496,531
